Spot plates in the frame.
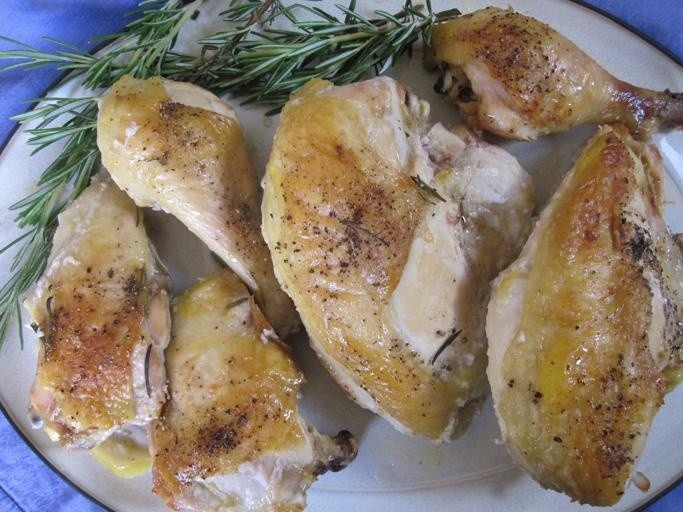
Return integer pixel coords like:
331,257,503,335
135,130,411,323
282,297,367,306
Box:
1,2,683,511
